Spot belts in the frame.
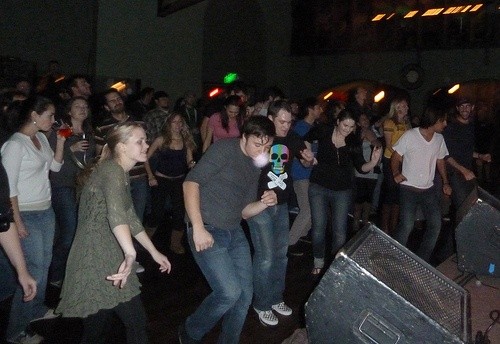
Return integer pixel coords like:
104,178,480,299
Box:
188,221,207,228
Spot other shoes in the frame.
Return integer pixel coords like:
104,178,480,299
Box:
272,301,293,316
7,329,44,344
254,308,278,326
288,246,303,256
29,307,61,323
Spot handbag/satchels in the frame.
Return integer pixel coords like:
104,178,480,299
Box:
329,168,350,191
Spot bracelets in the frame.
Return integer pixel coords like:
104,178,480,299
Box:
478,153,481,159
444,183,448,186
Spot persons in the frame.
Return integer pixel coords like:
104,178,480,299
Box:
0,61,491,290
304,109,383,275
55,122,172,344
0,94,66,344
246,99,314,324
177,115,278,344
390,104,452,263
0,164,36,302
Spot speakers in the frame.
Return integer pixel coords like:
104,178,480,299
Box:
453,185,500,276
302,219,473,344
